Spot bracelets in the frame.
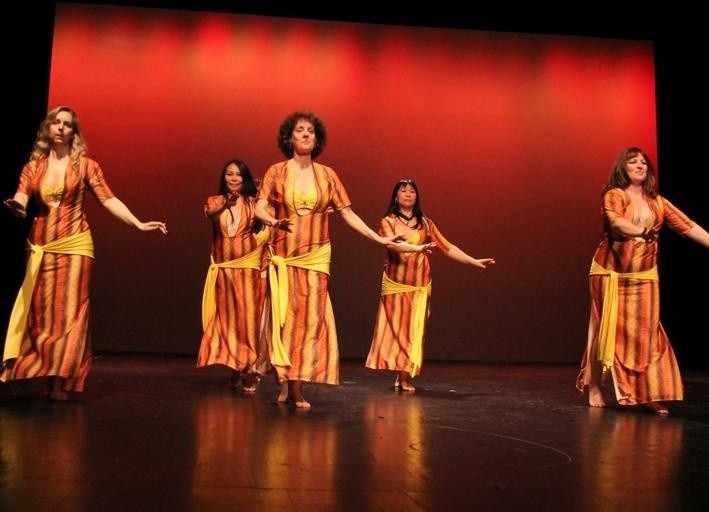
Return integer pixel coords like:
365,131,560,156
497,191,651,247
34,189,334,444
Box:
641,226,647,237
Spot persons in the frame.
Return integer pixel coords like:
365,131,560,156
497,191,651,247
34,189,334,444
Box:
194,159,270,393
574,145,709,416
1,103,169,403
254,110,406,410
363,178,497,392
